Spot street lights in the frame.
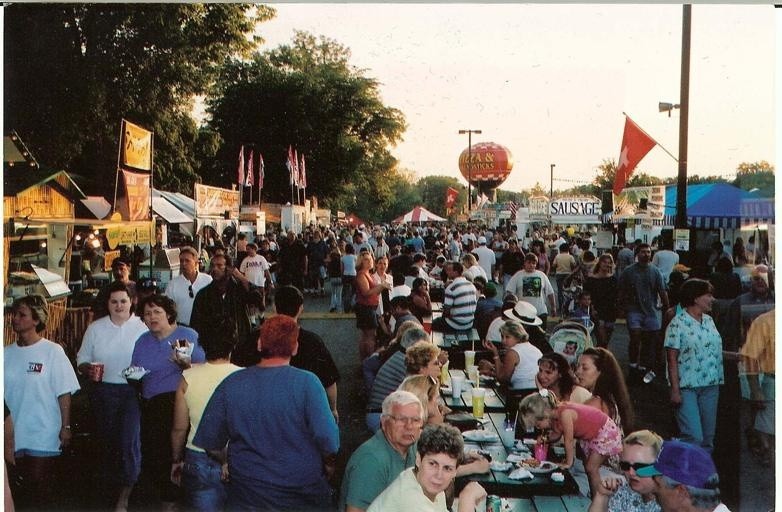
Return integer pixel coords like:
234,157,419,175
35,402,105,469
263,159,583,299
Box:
456,127,487,218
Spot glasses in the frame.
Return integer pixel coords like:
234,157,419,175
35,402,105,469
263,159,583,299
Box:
426,358,440,365
618,458,657,472
386,413,424,428
138,279,156,289
189,284,194,298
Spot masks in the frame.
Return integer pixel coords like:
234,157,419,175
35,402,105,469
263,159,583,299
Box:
682,272,690,280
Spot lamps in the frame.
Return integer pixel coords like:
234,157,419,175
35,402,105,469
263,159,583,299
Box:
659,102,679,117
59,229,101,267
16,206,33,224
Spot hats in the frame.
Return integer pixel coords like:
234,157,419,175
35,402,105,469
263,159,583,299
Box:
673,264,691,272
111,257,131,267
478,236,486,245
503,300,543,326
635,440,720,489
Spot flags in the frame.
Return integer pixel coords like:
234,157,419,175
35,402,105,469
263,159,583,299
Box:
293,149,300,185
612,115,657,197
244,149,255,187
285,145,294,185
444,187,457,208
299,152,308,190
239,145,245,184
258,154,265,187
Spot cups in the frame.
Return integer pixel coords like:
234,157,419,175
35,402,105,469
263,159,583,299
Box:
91,362,103,382
472,388,485,418
451,377,462,399
504,422,516,447
465,351,475,372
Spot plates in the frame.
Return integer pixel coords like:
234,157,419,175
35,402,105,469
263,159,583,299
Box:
521,461,557,472
462,430,499,442
447,413,475,420
533,443,549,461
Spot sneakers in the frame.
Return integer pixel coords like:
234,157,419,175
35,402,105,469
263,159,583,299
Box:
630,362,656,384
303,288,325,297
251,315,265,330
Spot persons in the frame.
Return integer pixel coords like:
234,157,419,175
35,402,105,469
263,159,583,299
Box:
2,219,775,512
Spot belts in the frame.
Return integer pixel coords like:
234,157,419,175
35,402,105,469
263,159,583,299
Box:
366,407,382,413
